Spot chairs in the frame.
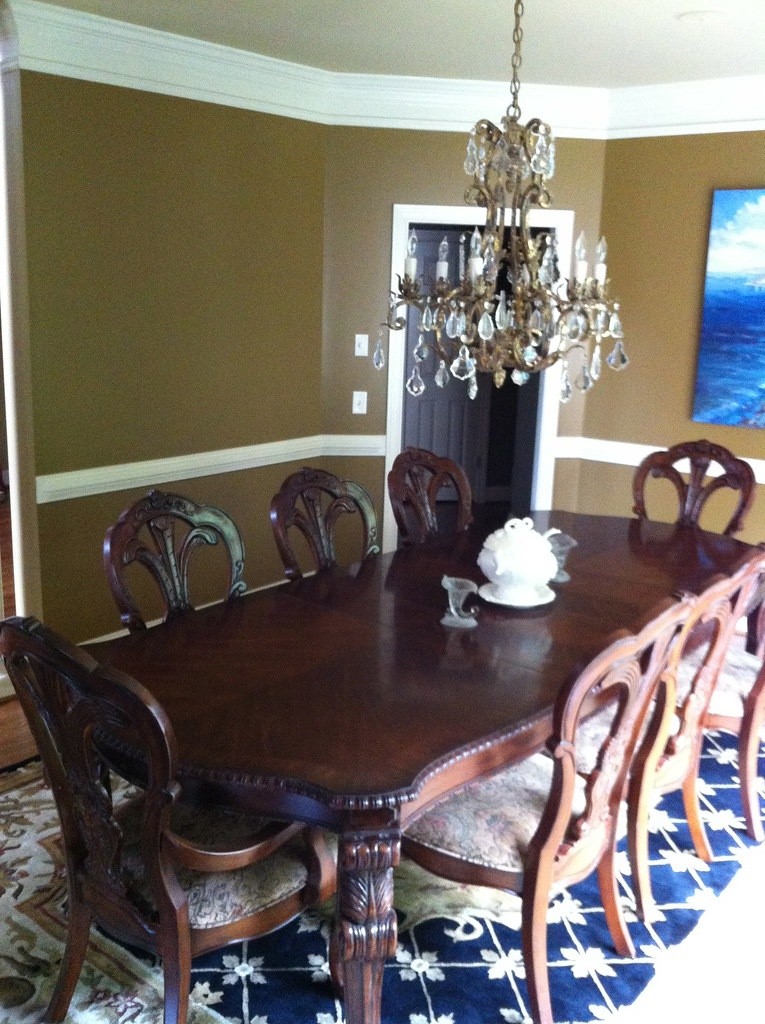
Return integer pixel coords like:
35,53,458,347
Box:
542,541,765,924
269,465,381,581
669,643,765,842
0,614,338,1024
401,590,698,1024
632,440,758,535
102,490,247,636
388,446,473,545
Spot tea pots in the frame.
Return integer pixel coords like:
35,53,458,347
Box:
477,516,558,593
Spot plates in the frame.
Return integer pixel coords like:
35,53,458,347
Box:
477,582,556,607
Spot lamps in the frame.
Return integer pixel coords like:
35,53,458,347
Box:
373,0,631,405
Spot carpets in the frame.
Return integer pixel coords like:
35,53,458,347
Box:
1,657,765,1024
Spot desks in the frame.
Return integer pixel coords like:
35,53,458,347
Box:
69,508,764,1024
